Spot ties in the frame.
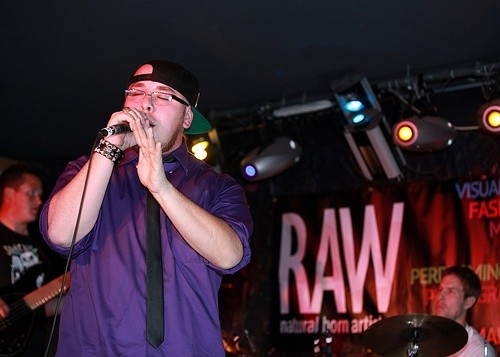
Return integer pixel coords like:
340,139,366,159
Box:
145,189,164,348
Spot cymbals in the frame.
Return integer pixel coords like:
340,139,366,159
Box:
361,312,469,357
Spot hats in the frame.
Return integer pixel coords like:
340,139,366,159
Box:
128,61,211,135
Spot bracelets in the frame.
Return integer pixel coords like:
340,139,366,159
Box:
95,139,124,164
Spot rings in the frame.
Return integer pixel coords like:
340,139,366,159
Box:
122,107,131,112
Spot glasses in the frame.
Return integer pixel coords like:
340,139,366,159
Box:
124,89,192,110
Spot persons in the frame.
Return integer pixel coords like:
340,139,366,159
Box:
39,59,254,357
434,266,494,357
0,163,65,357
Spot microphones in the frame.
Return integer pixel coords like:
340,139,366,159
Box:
98,120,133,138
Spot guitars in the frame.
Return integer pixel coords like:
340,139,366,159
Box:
0,269,71,357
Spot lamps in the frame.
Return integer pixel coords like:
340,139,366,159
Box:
396,116,455,152
477,98,500,133
241,136,299,183
330,68,380,127
